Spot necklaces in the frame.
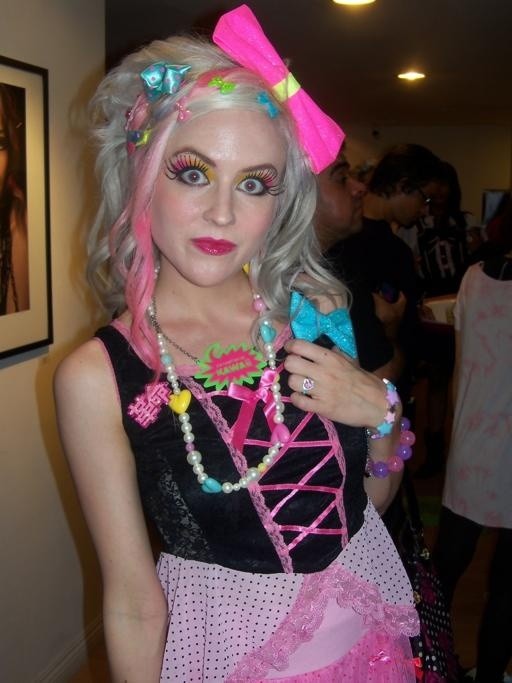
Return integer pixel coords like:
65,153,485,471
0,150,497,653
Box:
150,296,262,364
147,261,292,494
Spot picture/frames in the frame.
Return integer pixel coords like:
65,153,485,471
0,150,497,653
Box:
0,54,54,360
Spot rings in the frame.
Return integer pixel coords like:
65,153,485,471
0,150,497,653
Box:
303,377,314,395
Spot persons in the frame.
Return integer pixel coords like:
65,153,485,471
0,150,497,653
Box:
0,81,33,317
49,33,427,683
306,136,486,481
427,186,512,682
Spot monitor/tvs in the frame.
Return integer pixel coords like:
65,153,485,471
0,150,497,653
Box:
480,187,504,220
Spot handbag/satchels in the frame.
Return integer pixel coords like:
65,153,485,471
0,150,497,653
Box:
400,546,462,683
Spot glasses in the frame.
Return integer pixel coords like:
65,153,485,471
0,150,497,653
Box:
416,185,433,205
